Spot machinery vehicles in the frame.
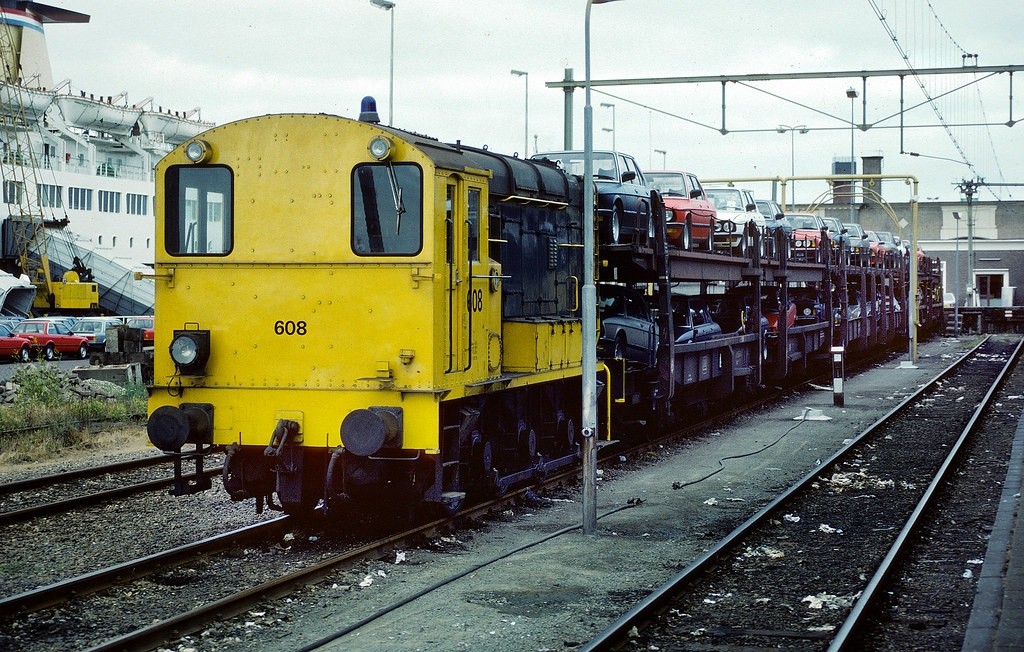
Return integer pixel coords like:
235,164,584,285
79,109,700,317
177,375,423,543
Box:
0,15,100,312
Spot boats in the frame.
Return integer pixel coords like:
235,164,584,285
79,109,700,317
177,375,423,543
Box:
54,94,144,131
1,80,57,117
140,111,216,144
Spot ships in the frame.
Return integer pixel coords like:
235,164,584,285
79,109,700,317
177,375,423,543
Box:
1,1,223,316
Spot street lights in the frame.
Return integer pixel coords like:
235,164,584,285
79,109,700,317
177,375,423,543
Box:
513,67,528,160
655,148,668,169
952,210,961,337
847,87,857,224
582,1,620,534
374,0,397,130
777,122,809,210
601,101,616,149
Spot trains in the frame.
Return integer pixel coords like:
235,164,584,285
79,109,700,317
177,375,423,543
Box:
144,96,945,553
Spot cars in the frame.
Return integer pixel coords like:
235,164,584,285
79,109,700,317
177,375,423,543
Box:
532,150,926,272
601,284,901,377
0,316,160,364
